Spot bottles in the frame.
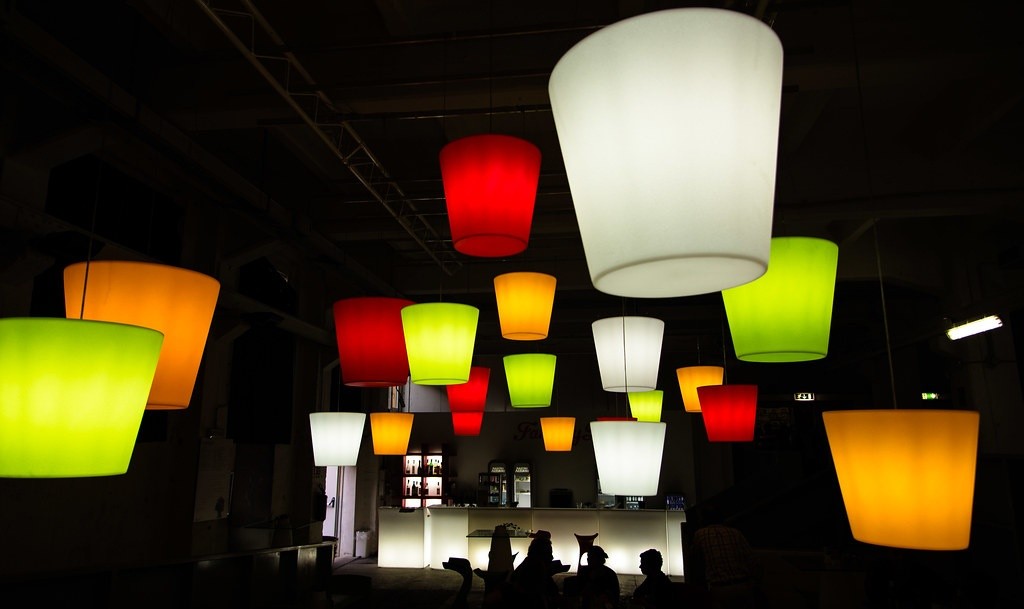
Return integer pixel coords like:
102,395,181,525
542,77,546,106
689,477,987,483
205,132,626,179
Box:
405,459,442,496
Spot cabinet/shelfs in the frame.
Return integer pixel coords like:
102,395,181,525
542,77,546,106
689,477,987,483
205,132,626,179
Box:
396,445,449,510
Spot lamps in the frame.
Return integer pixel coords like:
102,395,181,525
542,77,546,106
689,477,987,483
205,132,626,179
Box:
308,8,981,551
0,259,220,481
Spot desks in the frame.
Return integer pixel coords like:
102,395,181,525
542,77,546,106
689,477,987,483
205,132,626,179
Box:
465,527,531,540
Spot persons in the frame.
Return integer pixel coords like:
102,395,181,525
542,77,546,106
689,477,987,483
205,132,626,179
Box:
575,546,620,609
510,538,563,609
633,549,676,609
